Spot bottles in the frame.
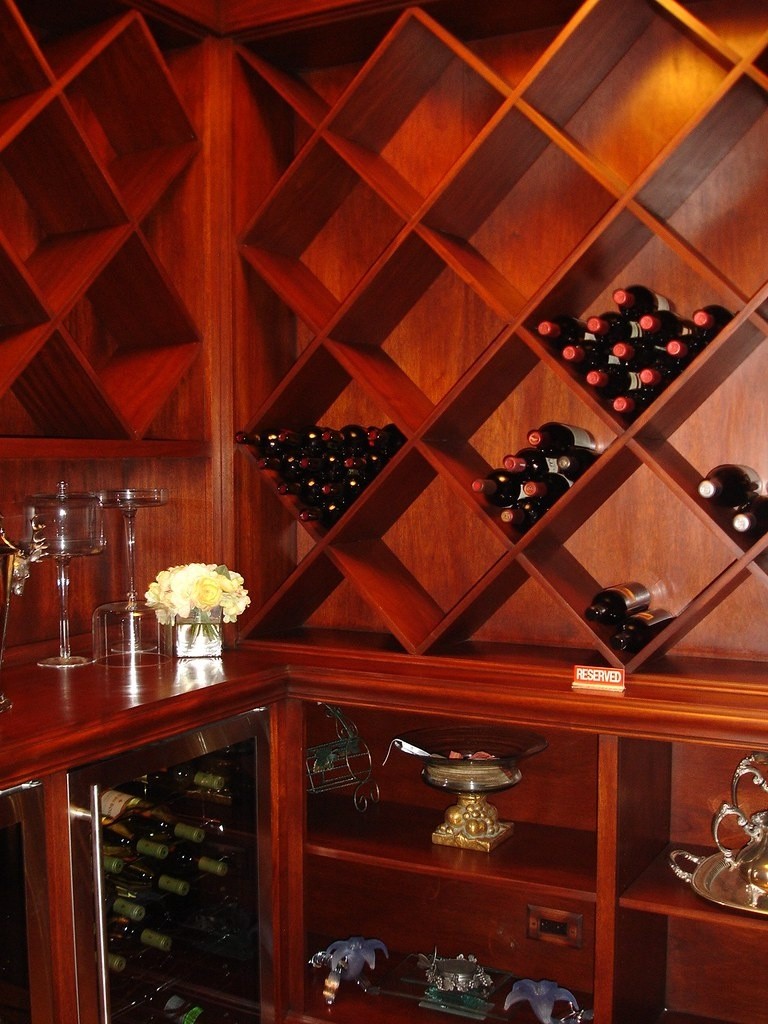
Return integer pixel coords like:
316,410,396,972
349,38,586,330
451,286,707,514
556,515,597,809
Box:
698,464,763,509
97,750,231,971
733,494,768,536
235,424,407,532
611,608,674,654
538,285,737,422
585,581,651,626
471,422,603,532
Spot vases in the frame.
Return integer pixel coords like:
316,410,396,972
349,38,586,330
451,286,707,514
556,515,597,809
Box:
176,606,224,657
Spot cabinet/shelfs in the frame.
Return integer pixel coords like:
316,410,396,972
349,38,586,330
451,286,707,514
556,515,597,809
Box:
0,0,215,459
0,617,768,1024
232,0,768,682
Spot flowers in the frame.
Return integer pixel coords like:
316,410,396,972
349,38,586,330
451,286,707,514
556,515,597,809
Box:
144,561,251,645
